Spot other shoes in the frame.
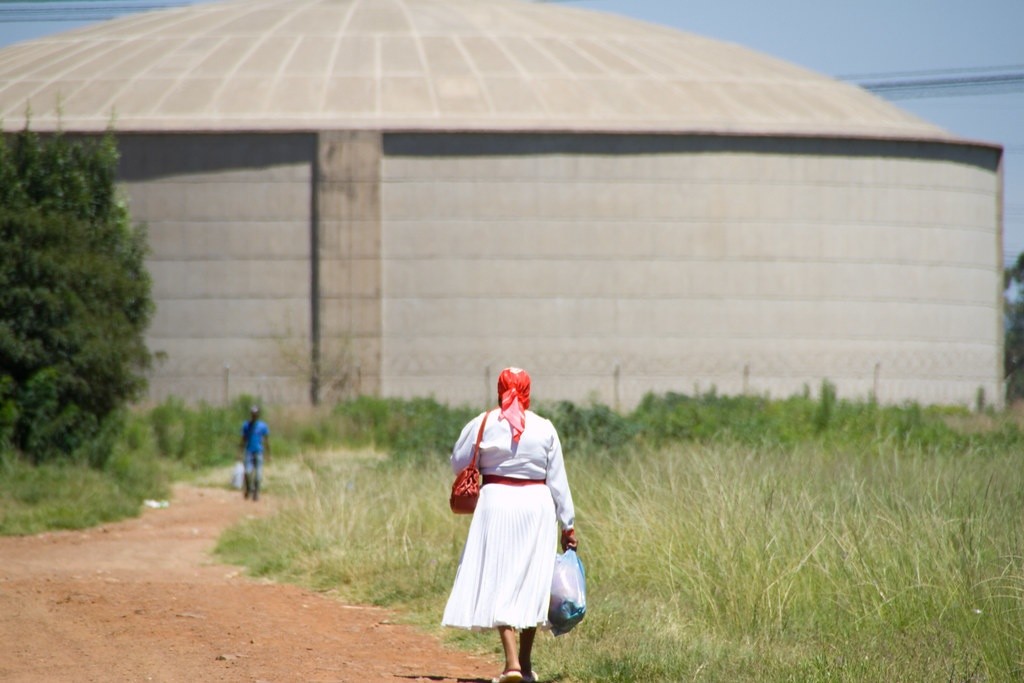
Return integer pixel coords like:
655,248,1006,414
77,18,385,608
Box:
492,671,522,683
253,489,259,501
521,671,538,683
244,489,252,498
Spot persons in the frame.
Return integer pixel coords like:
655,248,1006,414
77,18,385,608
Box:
448,367,577,683
238,406,272,501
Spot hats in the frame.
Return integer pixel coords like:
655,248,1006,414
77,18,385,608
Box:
250,406,259,415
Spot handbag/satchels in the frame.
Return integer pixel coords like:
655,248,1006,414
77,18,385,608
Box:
545,548,586,636
232,461,244,489
450,410,491,514
